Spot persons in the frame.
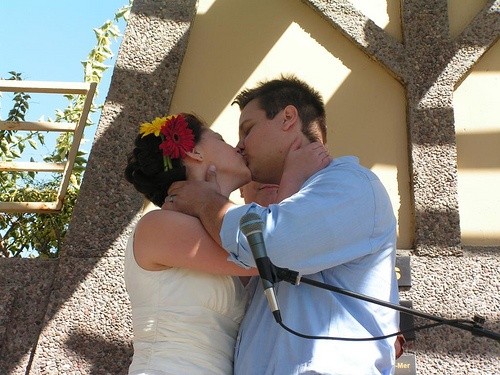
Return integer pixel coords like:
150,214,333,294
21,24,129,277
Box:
162,72,400,375
124,113,331,375
240,181,279,207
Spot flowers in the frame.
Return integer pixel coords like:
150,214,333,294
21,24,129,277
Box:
139,113,195,173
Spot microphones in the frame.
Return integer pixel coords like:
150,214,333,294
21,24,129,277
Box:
239,213,281,323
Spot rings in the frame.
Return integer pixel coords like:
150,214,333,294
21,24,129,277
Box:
169,195,176,203
